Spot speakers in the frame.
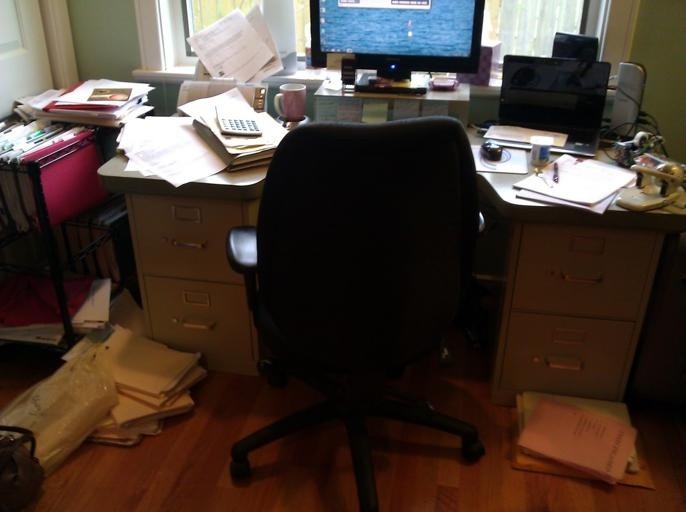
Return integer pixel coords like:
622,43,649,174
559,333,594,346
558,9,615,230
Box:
609,60,647,141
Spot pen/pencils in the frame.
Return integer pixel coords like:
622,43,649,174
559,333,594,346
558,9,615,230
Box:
552,161,559,184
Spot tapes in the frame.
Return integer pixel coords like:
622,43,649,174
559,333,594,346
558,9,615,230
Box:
633,130,650,148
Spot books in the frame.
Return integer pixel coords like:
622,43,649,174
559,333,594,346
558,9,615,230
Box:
192,112,289,172
515,391,639,486
52,199,136,294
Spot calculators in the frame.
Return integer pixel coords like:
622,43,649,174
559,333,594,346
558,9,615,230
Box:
214,104,263,137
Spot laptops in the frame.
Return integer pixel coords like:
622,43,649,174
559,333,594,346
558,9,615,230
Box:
484,54,612,159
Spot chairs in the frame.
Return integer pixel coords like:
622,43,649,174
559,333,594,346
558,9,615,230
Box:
228,123,512,512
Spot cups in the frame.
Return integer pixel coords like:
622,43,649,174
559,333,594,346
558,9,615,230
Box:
529,135,555,167
273,83,307,122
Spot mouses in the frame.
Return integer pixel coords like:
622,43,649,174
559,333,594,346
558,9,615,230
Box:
480,141,503,160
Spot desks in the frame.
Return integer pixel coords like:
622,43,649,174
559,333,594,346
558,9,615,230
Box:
98,81,685,406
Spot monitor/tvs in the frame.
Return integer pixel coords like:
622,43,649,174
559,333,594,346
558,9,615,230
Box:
309,0,487,95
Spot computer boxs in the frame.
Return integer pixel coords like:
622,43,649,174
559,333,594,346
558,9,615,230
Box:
312,70,472,123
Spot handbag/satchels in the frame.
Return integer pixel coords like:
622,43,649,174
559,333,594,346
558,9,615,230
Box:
0,426,43,512
